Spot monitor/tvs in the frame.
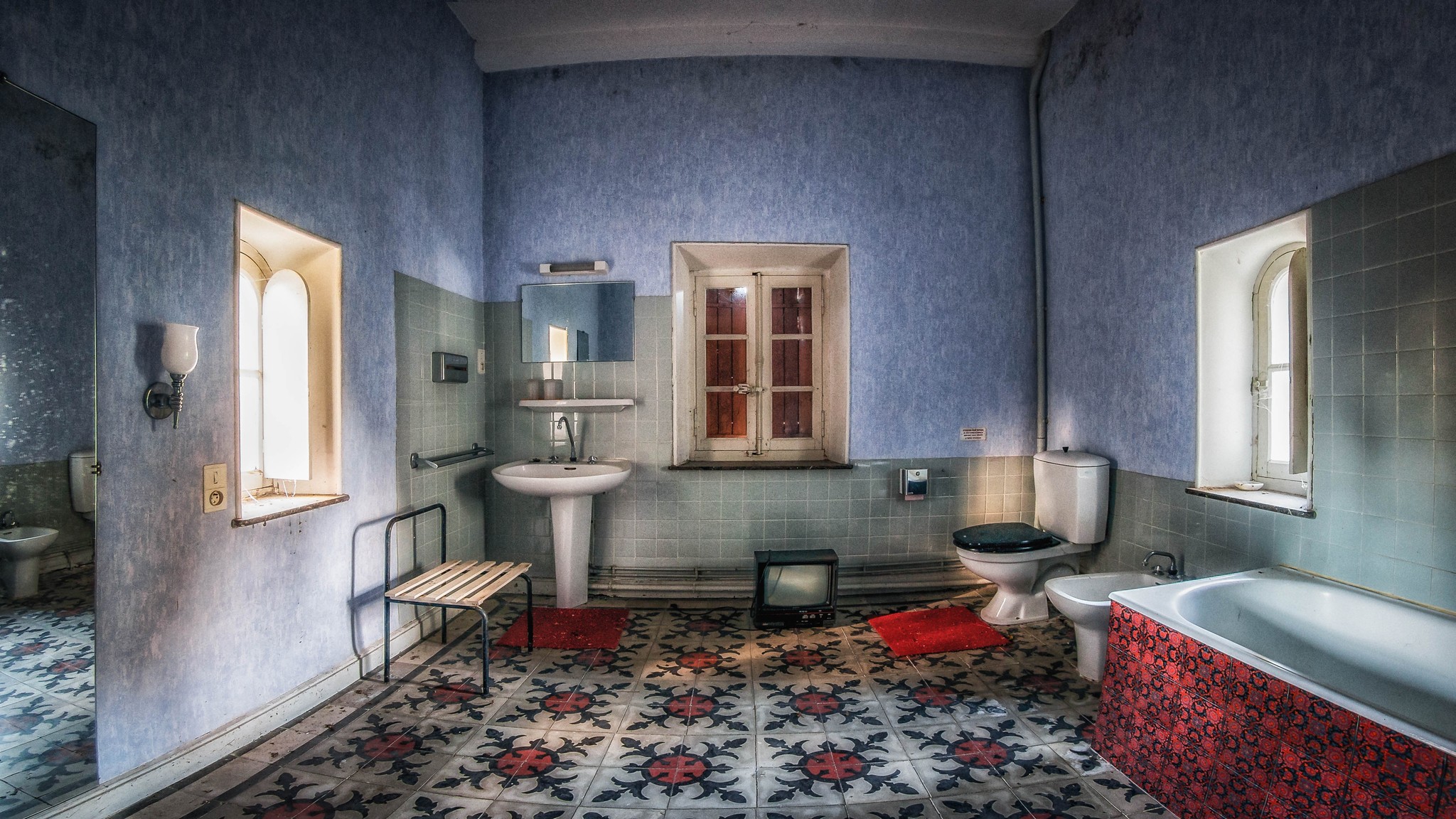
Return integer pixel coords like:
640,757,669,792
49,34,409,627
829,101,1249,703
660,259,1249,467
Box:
753,549,839,630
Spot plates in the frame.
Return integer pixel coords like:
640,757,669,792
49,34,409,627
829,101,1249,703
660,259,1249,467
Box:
1234,481,1264,491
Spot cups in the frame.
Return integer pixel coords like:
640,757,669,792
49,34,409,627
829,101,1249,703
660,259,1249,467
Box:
527,379,540,400
544,379,555,400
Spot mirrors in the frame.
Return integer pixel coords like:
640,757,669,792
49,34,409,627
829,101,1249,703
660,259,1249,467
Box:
518,278,637,366
1,77,109,819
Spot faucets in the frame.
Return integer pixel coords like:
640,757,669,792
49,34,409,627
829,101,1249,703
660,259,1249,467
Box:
557,416,579,462
1140,551,1179,580
2,510,20,529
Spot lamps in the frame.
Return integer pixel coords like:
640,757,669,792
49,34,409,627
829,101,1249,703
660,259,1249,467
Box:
138,318,202,434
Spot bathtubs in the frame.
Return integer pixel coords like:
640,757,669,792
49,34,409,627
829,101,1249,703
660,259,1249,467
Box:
1092,562,1456,819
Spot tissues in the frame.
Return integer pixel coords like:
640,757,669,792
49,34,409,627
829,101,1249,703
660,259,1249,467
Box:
898,467,928,502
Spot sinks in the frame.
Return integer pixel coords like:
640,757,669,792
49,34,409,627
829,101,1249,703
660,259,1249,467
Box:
1044,564,1191,626
492,457,633,497
0,523,60,559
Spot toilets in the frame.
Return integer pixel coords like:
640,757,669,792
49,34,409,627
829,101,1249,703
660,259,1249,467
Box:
67,450,95,522
953,446,1110,626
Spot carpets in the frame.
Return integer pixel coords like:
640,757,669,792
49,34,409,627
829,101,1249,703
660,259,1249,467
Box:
498,605,632,651
867,603,1012,660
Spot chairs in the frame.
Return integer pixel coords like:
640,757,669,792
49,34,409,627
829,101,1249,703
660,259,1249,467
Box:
379,501,536,701
